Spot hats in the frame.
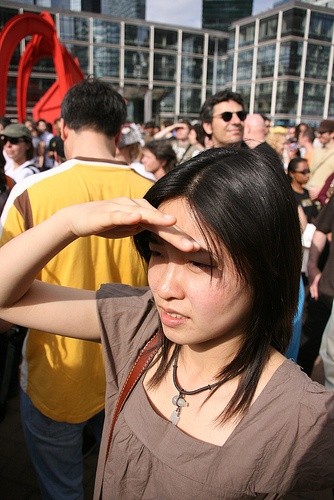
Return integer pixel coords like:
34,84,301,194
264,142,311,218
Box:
0,124,32,138
314,120,334,132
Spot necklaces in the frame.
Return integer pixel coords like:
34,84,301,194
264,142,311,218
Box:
170,345,248,427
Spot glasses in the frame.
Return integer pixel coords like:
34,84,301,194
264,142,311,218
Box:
296,169,310,174
3,138,25,145
213,111,247,122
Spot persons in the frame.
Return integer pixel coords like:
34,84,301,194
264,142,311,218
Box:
0,72,334,500
0,145,334,500
